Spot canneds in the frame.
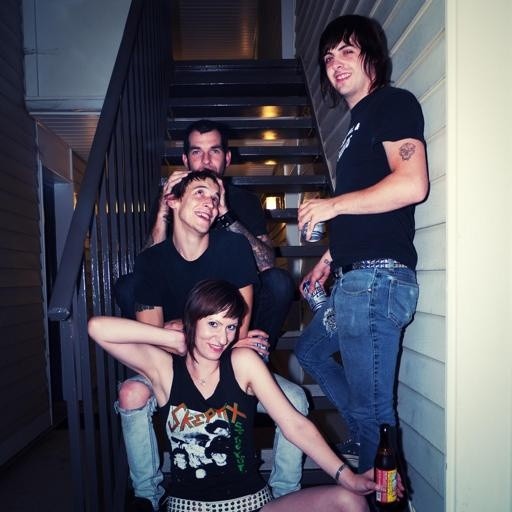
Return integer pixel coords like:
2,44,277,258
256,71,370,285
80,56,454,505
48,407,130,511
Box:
302,222,325,242
302,279,328,312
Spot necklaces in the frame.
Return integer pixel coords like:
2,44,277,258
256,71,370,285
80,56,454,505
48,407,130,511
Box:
189,365,219,386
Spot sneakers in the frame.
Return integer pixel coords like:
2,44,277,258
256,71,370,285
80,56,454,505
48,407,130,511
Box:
335,437,360,459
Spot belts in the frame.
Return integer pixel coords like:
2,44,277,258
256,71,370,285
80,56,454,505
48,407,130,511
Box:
335,258,408,278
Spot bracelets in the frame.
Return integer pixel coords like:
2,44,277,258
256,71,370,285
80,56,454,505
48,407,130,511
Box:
214,209,237,229
335,462,349,483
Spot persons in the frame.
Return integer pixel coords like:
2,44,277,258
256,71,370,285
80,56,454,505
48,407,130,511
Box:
115,118,295,354
294,11,432,480
86,276,406,512
114,169,310,511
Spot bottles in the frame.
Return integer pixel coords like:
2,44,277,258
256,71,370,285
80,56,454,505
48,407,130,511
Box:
373,422,399,512
